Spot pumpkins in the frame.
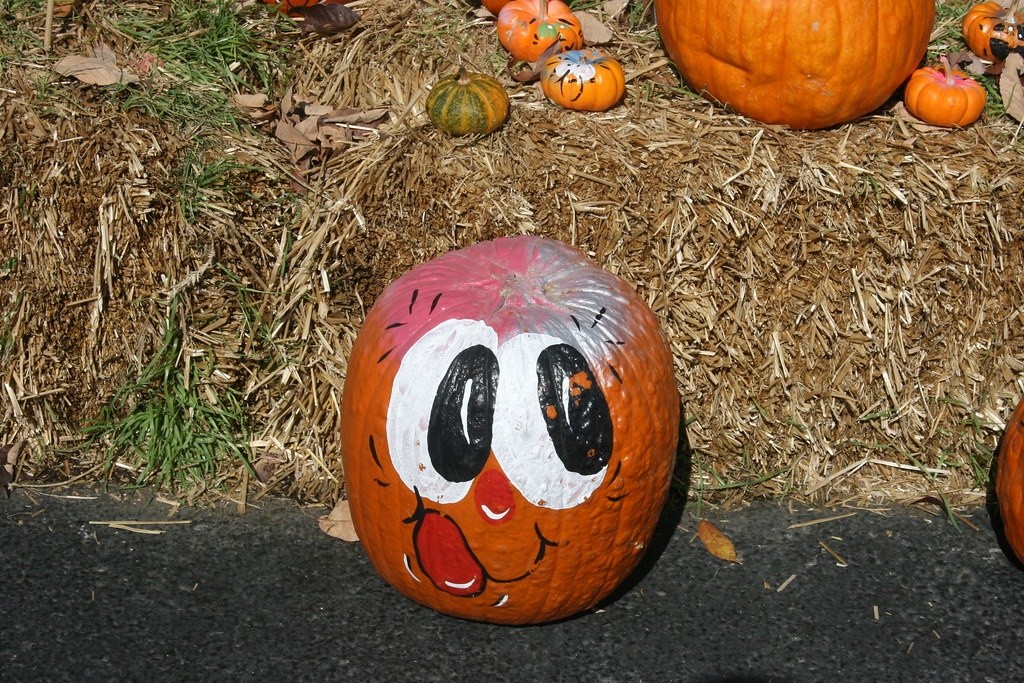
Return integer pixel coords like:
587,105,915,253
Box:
903,55,986,128
652,0,935,131
425,67,509,136
